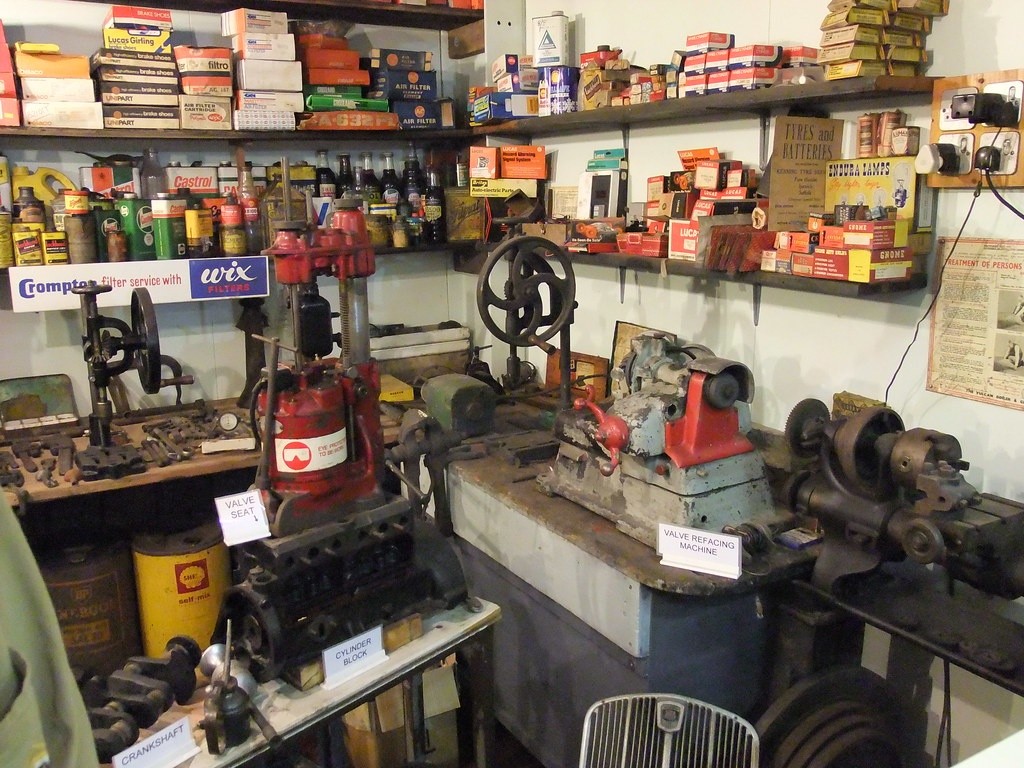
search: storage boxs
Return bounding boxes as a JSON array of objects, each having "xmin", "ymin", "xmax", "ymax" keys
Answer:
[
  {"xmin": 172, "ymin": 44, "xmax": 233, "ymax": 97},
  {"xmin": 469, "ymin": 145, "xmax": 501, "ymax": 179},
  {"xmin": 221, "ymin": 8, "xmax": 304, "ymax": 131},
  {"xmin": 577, "ymin": 148, "xmax": 628, "ymax": 218},
  {"xmin": 492, "ymin": 53, "xmax": 539, "ymax": 91},
  {"xmin": 21, "ymin": 100, "xmax": 105, "ymax": 129},
  {"xmin": 470, "ymin": 178, "xmax": 537, "ymax": 198},
  {"xmin": 218, "ymin": 167, "xmax": 267, "ymax": 198},
  {"xmin": 611, "ymin": 32, "xmax": 823, "ymax": 108},
  {"xmin": 20, "ymin": 78, "xmax": 96, "ymax": 101},
  {"xmin": 89, "ymin": 5, "xmax": 179, "ymax": 129},
  {"xmin": 816, "ymin": 0, "xmax": 949, "ymax": 81},
  {"xmin": 582, "ymin": 70, "xmax": 609, "ymax": 110},
  {"xmin": 761, "ymin": 156, "xmax": 931, "ymax": 285},
  {"xmin": 296, "ymin": 34, "xmax": 399, "ymax": 129},
  {"xmin": 370, "ymin": 324, "xmax": 471, "ymax": 382},
  {"xmin": 266, "ymin": 166, "xmax": 317, "ymax": 197},
  {"xmin": 521, "ymin": 220, "xmax": 596, "ymax": 245},
  {"xmin": 580, "ymin": 48, "xmax": 622, "ymax": 69},
  {"xmin": 179, "ymin": 94, "xmax": 232, "ymax": 130},
  {"xmin": 0, "ymin": 0, "xmax": 943, "ymax": 325},
  {"xmin": 616, "ymin": 147, "xmax": 757, "ymax": 261},
  {"xmin": 474, "ymin": 92, "xmax": 538, "ymax": 124},
  {"xmin": 328, "ymin": 653, "xmax": 461, "ymax": 768},
  {"xmin": 0, "ymin": 18, "xmax": 20, "ymax": 127},
  {"xmin": 78, "ymin": 166, "xmax": 219, "ymax": 199},
  {"xmin": 361, "ymin": 49, "xmax": 455, "ymax": 129},
  {"xmin": 396, "ymin": 0, "xmax": 484, "ymax": 9},
  {"xmin": 548, "ymin": 186, "xmax": 577, "ymax": 219},
  {"xmin": 15, "ymin": 51, "xmax": 90, "ymax": 78}
]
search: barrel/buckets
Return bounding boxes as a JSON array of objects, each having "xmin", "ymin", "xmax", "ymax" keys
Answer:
[
  {"xmin": 130, "ymin": 513, "xmax": 235, "ymax": 688},
  {"xmin": 33, "ymin": 533, "xmax": 143, "ymax": 683}
]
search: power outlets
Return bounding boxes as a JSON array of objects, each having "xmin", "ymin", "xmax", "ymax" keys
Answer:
[
  {"xmin": 940, "ymin": 87, "xmax": 978, "ymax": 130},
  {"xmin": 937, "ymin": 133, "xmax": 974, "ymax": 176},
  {"xmin": 977, "ymin": 130, "xmax": 1021, "ymax": 175}
]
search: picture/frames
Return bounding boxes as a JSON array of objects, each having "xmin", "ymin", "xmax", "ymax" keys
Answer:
[{"xmin": 926, "ymin": 237, "xmax": 1024, "ymax": 412}]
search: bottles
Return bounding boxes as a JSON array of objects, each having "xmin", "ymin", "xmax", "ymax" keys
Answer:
[
  {"xmin": 307, "ymin": 147, "xmax": 472, "ymax": 249},
  {"xmin": 0, "ymin": 146, "xmax": 306, "ymax": 274}
]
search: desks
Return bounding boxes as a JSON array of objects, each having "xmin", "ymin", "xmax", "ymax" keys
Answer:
[{"xmin": 136, "ymin": 596, "xmax": 502, "ymax": 768}]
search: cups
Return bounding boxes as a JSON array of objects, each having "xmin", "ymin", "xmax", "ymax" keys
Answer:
[
  {"xmin": 107, "ymin": 230, "xmax": 128, "ymax": 262},
  {"xmin": 64, "ymin": 190, "xmax": 89, "ymax": 215},
  {"xmin": 311, "ymin": 195, "xmax": 333, "ymax": 230}
]
